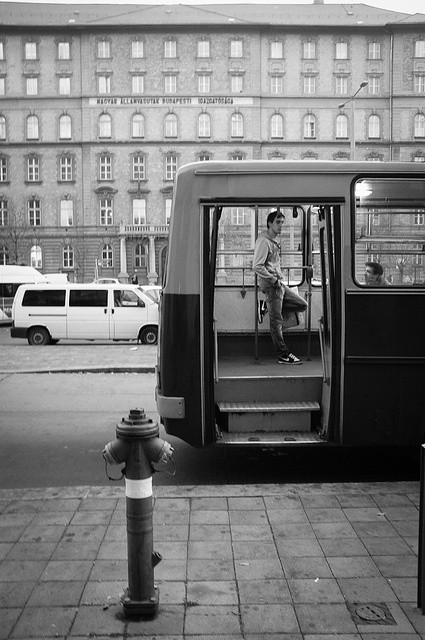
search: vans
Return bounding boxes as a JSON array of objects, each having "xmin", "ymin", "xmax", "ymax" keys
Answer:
[
  {"xmin": 121, "ymin": 285, "xmax": 162, "ymax": 306},
  {"xmin": 10, "ymin": 284, "xmax": 159, "ymax": 346}
]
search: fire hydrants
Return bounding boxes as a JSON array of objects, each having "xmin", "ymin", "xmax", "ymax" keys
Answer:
[{"xmin": 101, "ymin": 408, "xmax": 176, "ymax": 621}]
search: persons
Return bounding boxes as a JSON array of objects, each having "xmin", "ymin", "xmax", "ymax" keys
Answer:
[
  {"xmin": 363, "ymin": 260, "xmax": 393, "ymax": 287},
  {"xmin": 252, "ymin": 212, "xmax": 307, "ymax": 365}
]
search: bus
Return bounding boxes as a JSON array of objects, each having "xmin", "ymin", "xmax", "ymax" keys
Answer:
[
  {"xmin": 1, "ymin": 265, "xmax": 52, "ymax": 324},
  {"xmin": 155, "ymin": 160, "xmax": 425, "ymax": 454}
]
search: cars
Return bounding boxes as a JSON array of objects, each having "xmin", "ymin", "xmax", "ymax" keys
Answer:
[{"xmin": 91, "ymin": 277, "xmax": 121, "ymax": 284}]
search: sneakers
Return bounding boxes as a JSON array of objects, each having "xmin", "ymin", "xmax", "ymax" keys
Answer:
[
  {"xmin": 257, "ymin": 299, "xmax": 268, "ymax": 324},
  {"xmin": 276, "ymin": 352, "xmax": 303, "ymax": 365}
]
